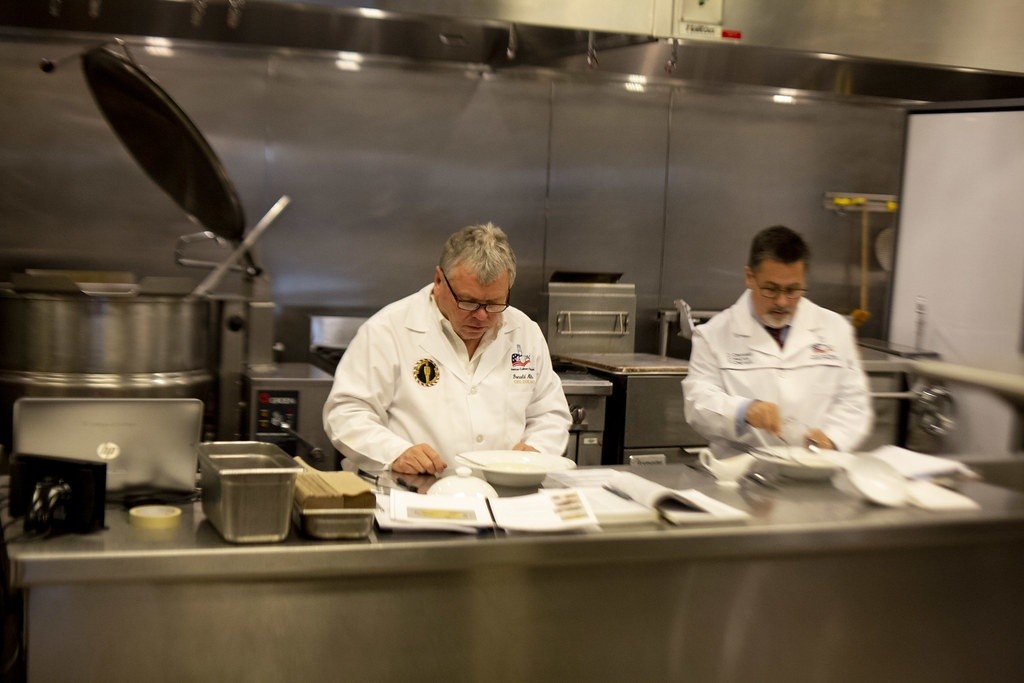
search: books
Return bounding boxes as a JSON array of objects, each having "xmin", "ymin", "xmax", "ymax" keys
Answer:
[{"xmin": 376, "ymin": 470, "xmax": 750, "ymax": 533}]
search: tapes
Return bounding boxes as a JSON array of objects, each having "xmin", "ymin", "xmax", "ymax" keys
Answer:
[{"xmin": 129, "ymin": 506, "xmax": 183, "ymax": 528}]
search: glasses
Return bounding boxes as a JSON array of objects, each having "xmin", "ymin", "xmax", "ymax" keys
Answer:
[
  {"xmin": 439, "ymin": 267, "xmax": 511, "ymax": 313},
  {"xmin": 751, "ymin": 268, "xmax": 809, "ymax": 299}
]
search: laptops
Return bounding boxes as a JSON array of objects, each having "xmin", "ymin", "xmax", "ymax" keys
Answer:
[{"xmin": 14, "ymin": 398, "xmax": 204, "ymax": 493}]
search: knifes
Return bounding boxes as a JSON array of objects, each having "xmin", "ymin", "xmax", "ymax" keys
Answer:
[{"xmin": 357, "ymin": 468, "xmax": 397, "ymax": 488}]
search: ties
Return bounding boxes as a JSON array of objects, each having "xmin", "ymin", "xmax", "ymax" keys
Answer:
[{"xmin": 763, "ymin": 325, "xmax": 785, "ymax": 348}]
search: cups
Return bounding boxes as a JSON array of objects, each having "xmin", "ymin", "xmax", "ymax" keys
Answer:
[{"xmin": 698, "ymin": 449, "xmax": 754, "ymax": 487}]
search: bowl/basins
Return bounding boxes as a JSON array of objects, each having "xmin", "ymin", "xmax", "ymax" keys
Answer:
[
  {"xmin": 454, "ymin": 450, "xmax": 576, "ymax": 486},
  {"xmin": 750, "ymin": 445, "xmax": 839, "ymax": 481}
]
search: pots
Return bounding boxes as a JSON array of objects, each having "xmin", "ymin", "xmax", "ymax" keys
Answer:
[{"xmin": 0, "ymin": 290, "xmax": 220, "ymax": 472}]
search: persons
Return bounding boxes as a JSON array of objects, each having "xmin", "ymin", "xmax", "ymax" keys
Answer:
[
  {"xmin": 682, "ymin": 226, "xmax": 875, "ymax": 460},
  {"xmin": 321, "ymin": 220, "xmax": 575, "ymax": 481}
]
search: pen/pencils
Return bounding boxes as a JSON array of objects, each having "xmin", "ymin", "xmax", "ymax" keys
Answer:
[{"xmin": 602, "ymin": 483, "xmax": 629, "ymax": 502}]
